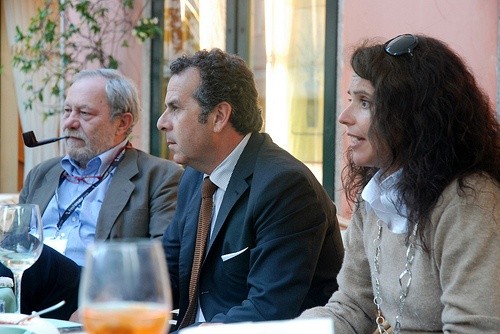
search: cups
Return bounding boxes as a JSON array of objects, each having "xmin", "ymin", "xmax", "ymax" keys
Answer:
[{"xmin": 78, "ymin": 238, "xmax": 172, "ymax": 334}]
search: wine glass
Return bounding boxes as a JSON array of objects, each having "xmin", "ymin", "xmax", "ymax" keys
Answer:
[{"xmin": 0, "ymin": 203, "xmax": 44, "ymax": 314}]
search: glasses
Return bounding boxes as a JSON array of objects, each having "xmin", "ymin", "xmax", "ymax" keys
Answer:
[
  {"xmin": 384, "ymin": 33, "xmax": 418, "ymax": 62},
  {"xmin": 62, "ymin": 145, "xmax": 130, "ymax": 185}
]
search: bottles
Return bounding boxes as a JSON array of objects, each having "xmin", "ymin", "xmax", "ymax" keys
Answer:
[{"xmin": 0, "ymin": 276, "xmax": 16, "ymax": 314}]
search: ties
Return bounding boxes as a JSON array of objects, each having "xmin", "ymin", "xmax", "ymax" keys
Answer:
[{"xmin": 172, "ymin": 176, "xmax": 219, "ymax": 330}]
